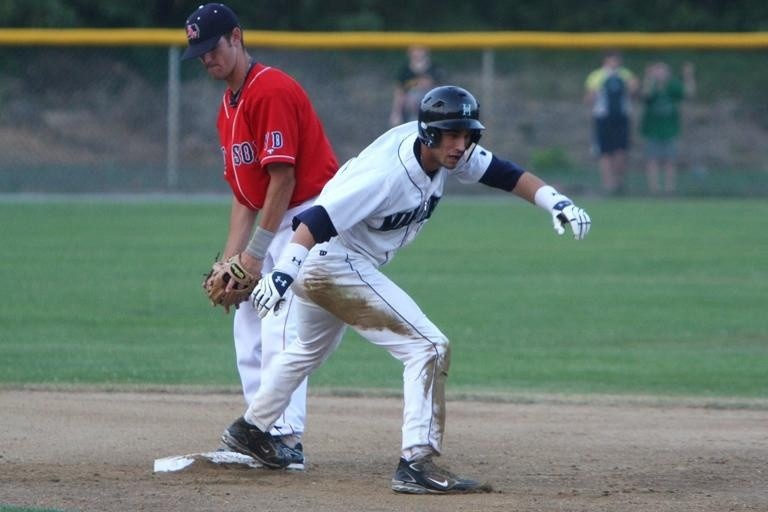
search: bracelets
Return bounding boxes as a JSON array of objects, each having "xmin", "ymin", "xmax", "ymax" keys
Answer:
[{"xmin": 243, "ymin": 224, "xmax": 274, "ymax": 261}]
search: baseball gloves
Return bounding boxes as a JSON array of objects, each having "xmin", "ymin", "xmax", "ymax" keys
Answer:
[{"xmin": 204, "ymin": 254, "xmax": 261, "ymax": 307}]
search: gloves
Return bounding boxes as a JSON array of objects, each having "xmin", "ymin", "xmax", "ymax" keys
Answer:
[
  {"xmin": 250, "ymin": 243, "xmax": 310, "ymax": 319},
  {"xmin": 534, "ymin": 186, "xmax": 591, "ymax": 240}
]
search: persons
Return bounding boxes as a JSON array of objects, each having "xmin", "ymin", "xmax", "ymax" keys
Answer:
[
  {"xmin": 636, "ymin": 58, "xmax": 696, "ymax": 198},
  {"xmin": 581, "ymin": 49, "xmax": 642, "ymax": 198},
  {"xmin": 384, "ymin": 45, "xmax": 445, "ymax": 126},
  {"xmin": 215, "ymin": 82, "xmax": 592, "ymax": 498},
  {"xmin": 176, "ymin": 1, "xmax": 342, "ymax": 473}
]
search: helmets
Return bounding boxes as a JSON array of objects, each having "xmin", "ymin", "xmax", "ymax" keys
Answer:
[{"xmin": 418, "ymin": 85, "xmax": 487, "ymax": 149}]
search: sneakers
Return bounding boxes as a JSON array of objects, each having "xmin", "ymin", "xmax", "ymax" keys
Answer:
[
  {"xmin": 391, "ymin": 454, "xmax": 481, "ymax": 494},
  {"xmin": 208, "ymin": 415, "xmax": 305, "ymax": 471}
]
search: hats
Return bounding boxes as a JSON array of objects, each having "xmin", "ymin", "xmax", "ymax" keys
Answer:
[{"xmin": 180, "ymin": 2, "xmax": 240, "ymax": 62}]
